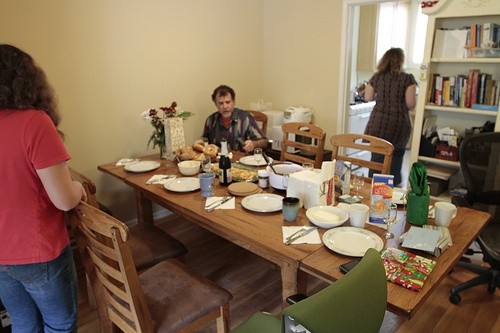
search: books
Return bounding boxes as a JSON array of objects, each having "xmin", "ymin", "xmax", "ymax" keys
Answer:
[
  {"xmin": 430, "ymin": 69, "xmax": 500, "ymax": 108},
  {"xmin": 471, "ymin": 22, "xmax": 500, "ymax": 49}
]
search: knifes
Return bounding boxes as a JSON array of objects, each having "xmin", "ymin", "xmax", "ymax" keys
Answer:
[
  {"xmin": 285, "ymin": 226, "xmax": 318, "ymax": 245},
  {"xmin": 207, "ymin": 196, "xmax": 232, "ymax": 212}
]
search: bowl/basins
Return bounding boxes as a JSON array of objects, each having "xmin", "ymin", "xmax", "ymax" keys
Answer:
[{"xmin": 178, "ymin": 160, "xmax": 200, "ymax": 176}]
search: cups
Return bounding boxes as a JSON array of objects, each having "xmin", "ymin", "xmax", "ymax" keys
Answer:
[
  {"xmin": 198, "ymin": 173, "xmax": 216, "ymax": 197},
  {"xmin": 349, "ymin": 203, "xmax": 369, "ymax": 228},
  {"xmin": 201, "ymin": 155, "xmax": 211, "ymax": 173},
  {"xmin": 266, "ymin": 162, "xmax": 303, "ymax": 190},
  {"xmin": 300, "ymin": 157, "xmax": 314, "ymax": 171},
  {"xmin": 434, "ymin": 202, "xmax": 458, "ymax": 227},
  {"xmin": 282, "ymin": 197, "xmax": 300, "ymax": 222}
]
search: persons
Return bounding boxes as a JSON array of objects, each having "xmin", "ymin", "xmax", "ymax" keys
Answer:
[
  {"xmin": 363, "ymin": 48, "xmax": 417, "ymax": 186},
  {"xmin": 203, "ymin": 86, "xmax": 266, "ymax": 153},
  {"xmin": 0, "ymin": 44, "xmax": 89, "ymax": 333}
]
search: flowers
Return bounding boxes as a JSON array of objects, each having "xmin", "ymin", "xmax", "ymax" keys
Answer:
[{"xmin": 146, "ymin": 101, "xmax": 195, "ymax": 148}]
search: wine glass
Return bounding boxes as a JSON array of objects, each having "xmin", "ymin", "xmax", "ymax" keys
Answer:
[
  {"xmin": 253, "ymin": 148, "xmax": 263, "ymax": 173},
  {"xmin": 353, "ymin": 172, "xmax": 365, "ymax": 201},
  {"xmin": 383, "ymin": 202, "xmax": 397, "ymax": 239}
]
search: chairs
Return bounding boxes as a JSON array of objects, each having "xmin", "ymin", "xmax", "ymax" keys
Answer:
[
  {"xmin": 280, "ymin": 122, "xmax": 327, "ymax": 169},
  {"xmin": 449, "ymin": 131, "xmax": 500, "ymax": 305},
  {"xmin": 233, "ymin": 248, "xmax": 388, "ymax": 333},
  {"xmin": 330, "ymin": 134, "xmax": 396, "ymax": 183},
  {"xmin": 67, "ymin": 168, "xmax": 186, "ymax": 308},
  {"xmin": 246, "ymin": 110, "xmax": 267, "ymax": 137},
  {"xmin": 76, "ymin": 202, "xmax": 233, "ymax": 333}
]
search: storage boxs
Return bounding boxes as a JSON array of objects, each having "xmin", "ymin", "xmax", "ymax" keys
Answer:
[
  {"xmin": 334, "ymin": 161, "xmax": 352, "ymax": 198},
  {"xmin": 370, "ymin": 173, "xmax": 395, "ymax": 224}
]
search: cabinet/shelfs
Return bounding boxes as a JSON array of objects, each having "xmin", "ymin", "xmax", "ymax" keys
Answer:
[{"xmin": 409, "ymin": 0, "xmax": 500, "ymax": 201}]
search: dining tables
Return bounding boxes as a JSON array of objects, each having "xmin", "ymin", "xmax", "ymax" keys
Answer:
[{"xmin": 97, "ymin": 150, "xmax": 492, "ymax": 312}]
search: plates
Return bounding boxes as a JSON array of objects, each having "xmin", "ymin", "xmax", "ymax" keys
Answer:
[
  {"xmin": 164, "ymin": 176, "xmax": 201, "ymax": 192},
  {"xmin": 241, "ymin": 193, "xmax": 285, "ymax": 213},
  {"xmin": 306, "ymin": 206, "xmax": 350, "ymax": 228},
  {"xmin": 124, "ymin": 161, "xmax": 160, "ymax": 172},
  {"xmin": 228, "ymin": 182, "xmax": 263, "ymax": 197},
  {"xmin": 322, "ymin": 226, "xmax": 384, "ymax": 257},
  {"xmin": 392, "ymin": 187, "xmax": 409, "ymax": 204},
  {"xmin": 240, "ymin": 155, "xmax": 272, "ymax": 165}
]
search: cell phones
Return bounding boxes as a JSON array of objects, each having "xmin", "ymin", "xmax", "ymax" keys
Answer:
[{"xmin": 339, "ymin": 257, "xmax": 363, "ymax": 275}]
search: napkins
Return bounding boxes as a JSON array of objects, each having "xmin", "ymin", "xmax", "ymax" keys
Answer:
[{"xmin": 406, "ymin": 161, "xmax": 430, "ymax": 225}]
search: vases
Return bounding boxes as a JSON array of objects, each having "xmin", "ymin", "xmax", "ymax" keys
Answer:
[{"xmin": 160, "ymin": 144, "xmax": 166, "ymax": 159}]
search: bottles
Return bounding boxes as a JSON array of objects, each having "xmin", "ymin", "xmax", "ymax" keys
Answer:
[{"xmin": 218, "ymin": 139, "xmax": 232, "ymax": 187}]
